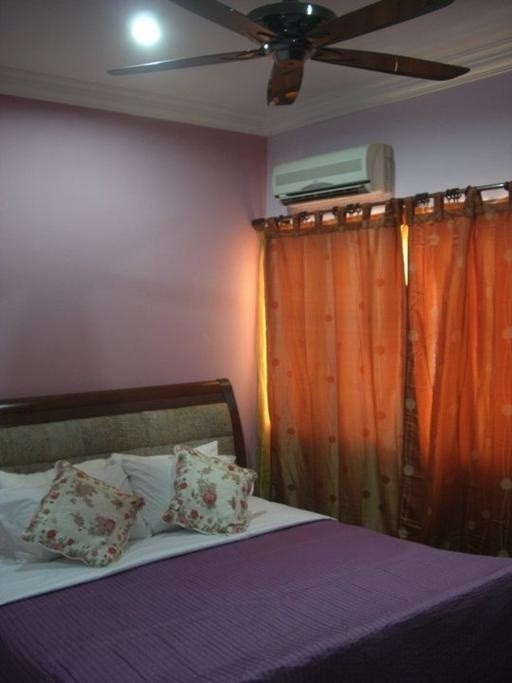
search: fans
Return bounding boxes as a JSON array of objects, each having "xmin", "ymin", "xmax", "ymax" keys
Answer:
[{"xmin": 107, "ymin": 0, "xmax": 471, "ymax": 109}]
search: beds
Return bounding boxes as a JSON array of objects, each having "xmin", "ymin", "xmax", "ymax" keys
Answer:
[{"xmin": 2, "ymin": 376, "xmax": 510, "ymax": 681}]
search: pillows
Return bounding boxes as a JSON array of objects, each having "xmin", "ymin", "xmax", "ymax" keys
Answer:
[{"xmin": 1, "ymin": 440, "xmax": 259, "ymax": 568}]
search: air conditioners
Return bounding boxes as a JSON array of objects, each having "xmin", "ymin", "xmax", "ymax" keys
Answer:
[{"xmin": 271, "ymin": 143, "xmax": 391, "ymax": 201}]
